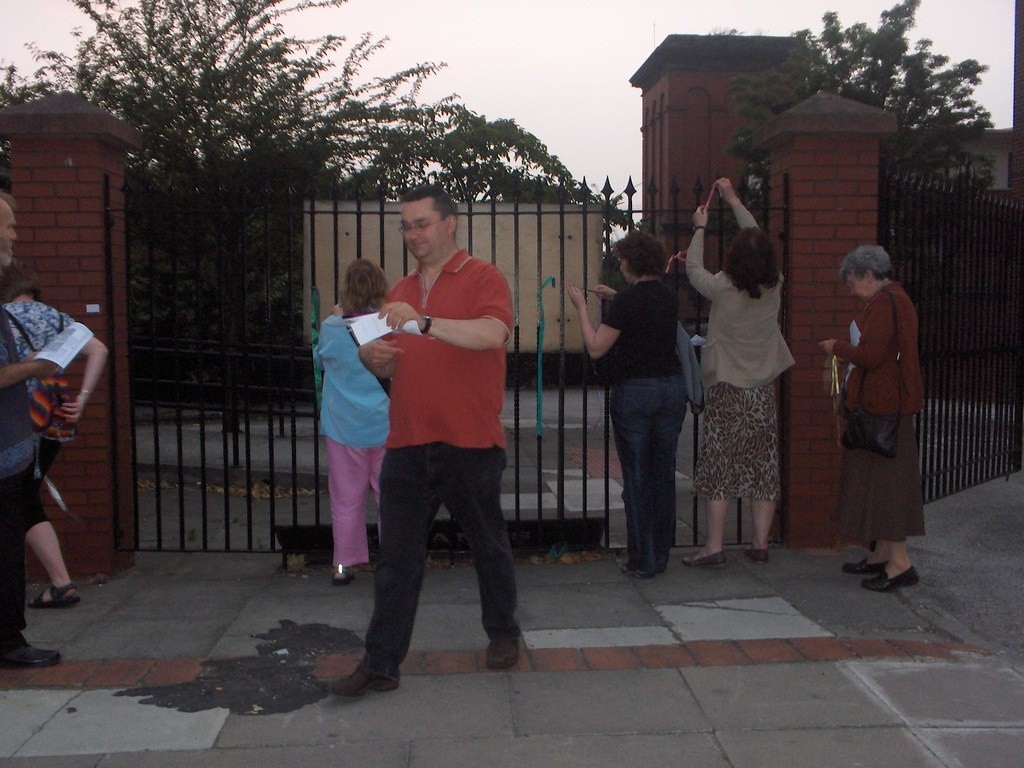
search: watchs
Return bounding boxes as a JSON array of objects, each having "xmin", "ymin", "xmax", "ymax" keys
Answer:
[{"xmin": 420, "ymin": 314, "xmax": 432, "ymax": 333}]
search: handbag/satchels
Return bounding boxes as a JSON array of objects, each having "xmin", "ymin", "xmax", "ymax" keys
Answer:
[{"xmin": 839, "ymin": 401, "xmax": 900, "ymax": 459}]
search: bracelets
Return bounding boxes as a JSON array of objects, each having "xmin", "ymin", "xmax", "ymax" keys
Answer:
[
  {"xmin": 692, "ymin": 225, "xmax": 705, "ymax": 235},
  {"xmin": 81, "ymin": 388, "xmax": 93, "ymax": 394}
]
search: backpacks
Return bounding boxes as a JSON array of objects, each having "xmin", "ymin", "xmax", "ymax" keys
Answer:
[{"xmin": 4, "ymin": 309, "xmax": 78, "ymax": 450}]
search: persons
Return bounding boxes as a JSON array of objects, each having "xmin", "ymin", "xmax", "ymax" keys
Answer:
[
  {"xmin": 332, "ymin": 184, "xmax": 521, "ymax": 697},
  {"xmin": 0, "ymin": 191, "xmax": 110, "ymax": 668},
  {"xmin": 819, "ymin": 243, "xmax": 927, "ymax": 593},
  {"xmin": 567, "ymin": 230, "xmax": 688, "ymax": 580},
  {"xmin": 681, "ymin": 177, "xmax": 796, "ymax": 568},
  {"xmin": 313, "ymin": 259, "xmax": 392, "ymax": 585}
]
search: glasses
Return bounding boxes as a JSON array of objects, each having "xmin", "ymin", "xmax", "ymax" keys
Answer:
[
  {"xmin": 399, "ymin": 218, "xmax": 446, "ymax": 234},
  {"xmin": 845, "ymin": 272, "xmax": 865, "ymax": 290}
]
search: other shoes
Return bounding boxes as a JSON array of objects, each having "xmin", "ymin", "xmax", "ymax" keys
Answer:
[
  {"xmin": 485, "ymin": 636, "xmax": 519, "ymax": 668},
  {"xmin": 683, "ymin": 550, "xmax": 728, "ymax": 568},
  {"xmin": 744, "ymin": 549, "xmax": 768, "ymax": 562},
  {"xmin": 332, "ymin": 665, "xmax": 400, "ymax": 697},
  {"xmin": 333, "ymin": 563, "xmax": 354, "ymax": 585},
  {"xmin": 622, "ymin": 563, "xmax": 665, "ymax": 578}
]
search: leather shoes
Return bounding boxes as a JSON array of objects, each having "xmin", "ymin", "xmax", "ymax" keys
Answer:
[
  {"xmin": 0, "ymin": 647, "xmax": 61, "ymax": 668},
  {"xmin": 842, "ymin": 556, "xmax": 888, "ymax": 574},
  {"xmin": 861, "ymin": 564, "xmax": 918, "ymax": 592}
]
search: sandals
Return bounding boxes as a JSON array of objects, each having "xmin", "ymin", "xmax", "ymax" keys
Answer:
[{"xmin": 27, "ymin": 582, "xmax": 82, "ymax": 609}]
